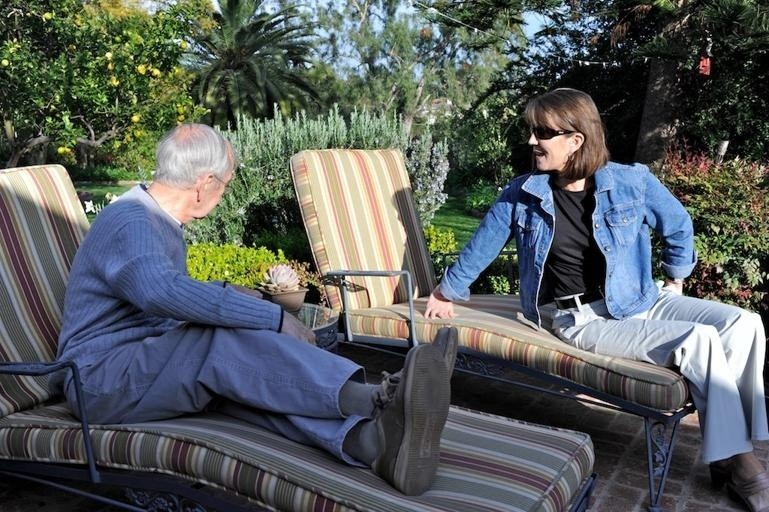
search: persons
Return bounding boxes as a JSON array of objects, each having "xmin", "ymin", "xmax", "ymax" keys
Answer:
[
  {"xmin": 421, "ymin": 86, "xmax": 769, "ymax": 511},
  {"xmin": 45, "ymin": 121, "xmax": 460, "ymax": 496}
]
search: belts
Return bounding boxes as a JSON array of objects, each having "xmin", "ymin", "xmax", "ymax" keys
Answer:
[{"xmin": 554, "ymin": 285, "xmax": 605, "ymax": 310}]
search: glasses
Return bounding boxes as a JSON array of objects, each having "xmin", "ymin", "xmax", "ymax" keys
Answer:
[
  {"xmin": 528, "ymin": 126, "xmax": 572, "ymax": 140},
  {"xmin": 214, "ymin": 174, "xmax": 234, "ymax": 195}
]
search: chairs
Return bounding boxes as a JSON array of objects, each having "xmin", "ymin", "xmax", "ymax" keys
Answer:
[
  {"xmin": 2, "ymin": 163, "xmax": 596, "ymax": 510},
  {"xmin": 288, "ymin": 149, "xmax": 696, "ymax": 510}
]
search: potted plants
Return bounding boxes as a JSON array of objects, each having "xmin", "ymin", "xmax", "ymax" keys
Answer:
[{"xmin": 256, "ymin": 262, "xmax": 309, "ymax": 312}]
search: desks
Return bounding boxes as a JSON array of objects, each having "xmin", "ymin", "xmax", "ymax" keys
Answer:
[{"xmin": 293, "ymin": 301, "xmax": 338, "ymax": 350}]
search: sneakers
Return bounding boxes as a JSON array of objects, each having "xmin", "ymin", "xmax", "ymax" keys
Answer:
[
  {"xmin": 370, "ymin": 344, "xmax": 452, "ymax": 496},
  {"xmin": 382, "ymin": 327, "xmax": 458, "ymax": 401}
]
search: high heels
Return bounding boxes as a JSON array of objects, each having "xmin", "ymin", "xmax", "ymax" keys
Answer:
[{"xmin": 709, "ymin": 459, "xmax": 769, "ymax": 512}]
